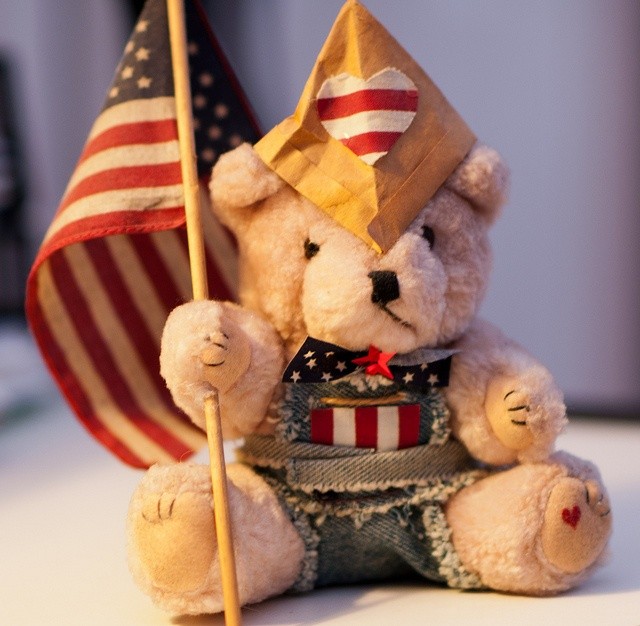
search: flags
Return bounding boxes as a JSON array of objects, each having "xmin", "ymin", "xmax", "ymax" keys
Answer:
[{"xmin": 25, "ymin": 0, "xmax": 267, "ymax": 475}]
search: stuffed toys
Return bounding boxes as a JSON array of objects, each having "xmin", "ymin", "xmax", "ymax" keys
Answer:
[{"xmin": 126, "ymin": 0, "xmax": 614, "ymax": 616}]
